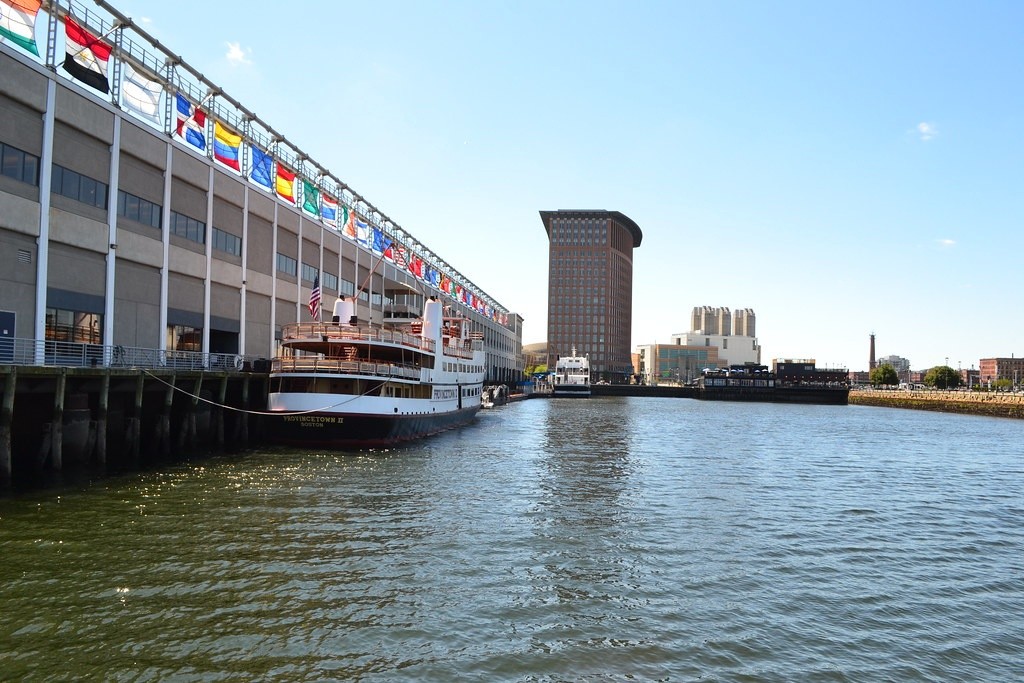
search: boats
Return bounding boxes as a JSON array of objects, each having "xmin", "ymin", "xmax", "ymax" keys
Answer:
[
  {"xmin": 553, "ymin": 346, "xmax": 591, "ymax": 399},
  {"xmin": 264, "ymin": 290, "xmax": 485, "ymax": 447}
]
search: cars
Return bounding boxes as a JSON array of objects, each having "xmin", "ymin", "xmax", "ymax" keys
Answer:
[
  {"xmin": 596, "ymin": 381, "xmax": 609, "ymax": 385},
  {"xmin": 850, "ymin": 382, "xmax": 1024, "ymax": 394}
]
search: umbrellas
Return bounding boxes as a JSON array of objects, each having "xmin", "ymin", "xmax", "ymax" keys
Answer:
[{"xmin": 703, "ymin": 366, "xmax": 768, "ymax": 374}]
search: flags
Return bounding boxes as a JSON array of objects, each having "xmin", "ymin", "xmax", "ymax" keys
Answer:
[
  {"xmin": 357, "ymin": 217, "xmax": 509, "ymax": 327},
  {"xmin": 251, "ymin": 144, "xmax": 274, "ymax": 189},
  {"xmin": 214, "ymin": 120, "xmax": 243, "ymax": 173},
  {"xmin": 62, "ymin": 14, "xmax": 116, "ymax": 96},
  {"xmin": 303, "ymin": 177, "xmax": 320, "ymax": 216},
  {"xmin": 1, "ymin": 0, "xmax": 42, "ymax": 57},
  {"xmin": 276, "ymin": 161, "xmax": 297, "ymax": 203},
  {"xmin": 341, "ymin": 204, "xmax": 356, "ymax": 237},
  {"xmin": 308, "ymin": 276, "xmax": 320, "ymax": 321},
  {"xmin": 322, "ymin": 194, "xmax": 338, "ymax": 222},
  {"xmin": 176, "ymin": 90, "xmax": 208, "ymax": 152},
  {"xmin": 119, "ymin": 60, "xmax": 164, "ymax": 127}
]
